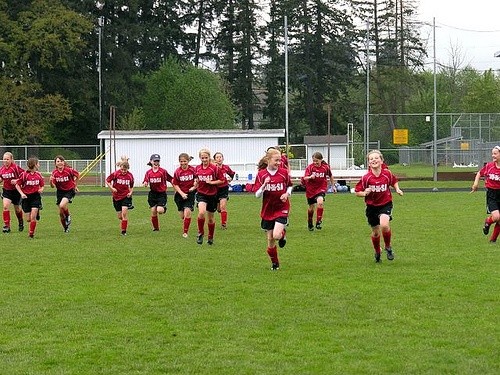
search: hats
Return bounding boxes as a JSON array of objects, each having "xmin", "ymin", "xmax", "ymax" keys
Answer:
[{"xmin": 150, "ymin": 154, "xmax": 160, "ymax": 161}]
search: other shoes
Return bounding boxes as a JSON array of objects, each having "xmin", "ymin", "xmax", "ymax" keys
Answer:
[{"xmin": 488, "ymin": 240, "xmax": 496, "ymax": 245}]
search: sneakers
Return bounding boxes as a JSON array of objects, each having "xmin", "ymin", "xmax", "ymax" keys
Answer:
[
  {"xmin": 30, "ymin": 233, "xmax": 33, "ymax": 237},
  {"xmin": 271, "ymin": 262, "xmax": 280, "ymax": 271},
  {"xmin": 316, "ymin": 221, "xmax": 322, "ymax": 230},
  {"xmin": 308, "ymin": 221, "xmax": 314, "ymax": 232},
  {"xmin": 152, "ymin": 228, "xmax": 159, "ymax": 232},
  {"xmin": 384, "ymin": 247, "xmax": 394, "ymax": 261},
  {"xmin": 124, "ymin": 233, "xmax": 127, "ymax": 235},
  {"xmin": 63, "ymin": 227, "xmax": 69, "ymax": 233},
  {"xmin": 208, "ymin": 239, "xmax": 213, "ymax": 245},
  {"xmin": 65, "ymin": 216, "xmax": 71, "ymax": 225},
  {"xmin": 279, "ymin": 228, "xmax": 287, "ymax": 248},
  {"xmin": 183, "ymin": 233, "xmax": 188, "ymax": 238},
  {"xmin": 483, "ymin": 217, "xmax": 490, "ymax": 236},
  {"xmin": 19, "ymin": 223, "xmax": 24, "ymax": 231},
  {"xmin": 374, "ymin": 254, "xmax": 382, "ymax": 264},
  {"xmin": 196, "ymin": 233, "xmax": 203, "ymax": 244},
  {"xmin": 37, "ymin": 216, "xmax": 40, "ymax": 220},
  {"xmin": 3, "ymin": 226, "xmax": 10, "ymax": 233},
  {"xmin": 220, "ymin": 224, "xmax": 226, "ymax": 230}
]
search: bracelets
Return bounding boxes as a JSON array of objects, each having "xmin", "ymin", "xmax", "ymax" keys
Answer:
[{"xmin": 332, "ymin": 186, "xmax": 335, "ymax": 189}]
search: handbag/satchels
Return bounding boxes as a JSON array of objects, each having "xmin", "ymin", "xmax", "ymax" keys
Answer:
[
  {"xmin": 292, "ymin": 184, "xmax": 306, "ymax": 192},
  {"xmin": 326, "ymin": 177, "xmax": 356, "ymax": 194},
  {"xmin": 227, "ymin": 185, "xmax": 243, "ymax": 192},
  {"xmin": 243, "ymin": 183, "xmax": 254, "ymax": 192}
]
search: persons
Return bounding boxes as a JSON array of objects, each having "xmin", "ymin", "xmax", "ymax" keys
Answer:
[
  {"xmin": 106, "ymin": 162, "xmax": 133, "ymax": 237},
  {"xmin": 195, "ymin": 148, "xmax": 225, "ymax": 245},
  {"xmin": 143, "ymin": 154, "xmax": 174, "ymax": 231},
  {"xmin": 15, "ymin": 158, "xmax": 45, "ymax": 238},
  {"xmin": 254, "ymin": 149, "xmax": 293, "ymax": 271},
  {"xmin": 259, "ymin": 146, "xmax": 290, "ymax": 226},
  {"xmin": 0, "ymin": 152, "xmax": 23, "ymax": 233},
  {"xmin": 470, "ymin": 146, "xmax": 500, "ymax": 243},
  {"xmin": 355, "ymin": 150, "xmax": 403, "ymax": 263},
  {"xmin": 174, "ymin": 153, "xmax": 197, "ymax": 237},
  {"xmin": 214, "ymin": 152, "xmax": 235, "ymax": 227},
  {"xmin": 50, "ymin": 155, "xmax": 79, "ymax": 232},
  {"xmin": 303, "ymin": 152, "xmax": 338, "ymax": 231},
  {"xmin": 117, "ymin": 155, "xmax": 135, "ymax": 209}
]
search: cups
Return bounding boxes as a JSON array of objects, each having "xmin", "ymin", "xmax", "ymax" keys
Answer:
[{"xmin": 249, "ymin": 174, "xmax": 252, "ymax": 180}]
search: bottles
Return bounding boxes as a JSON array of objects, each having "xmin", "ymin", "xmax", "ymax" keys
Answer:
[{"xmin": 235, "ymin": 174, "xmax": 238, "ymax": 180}]
